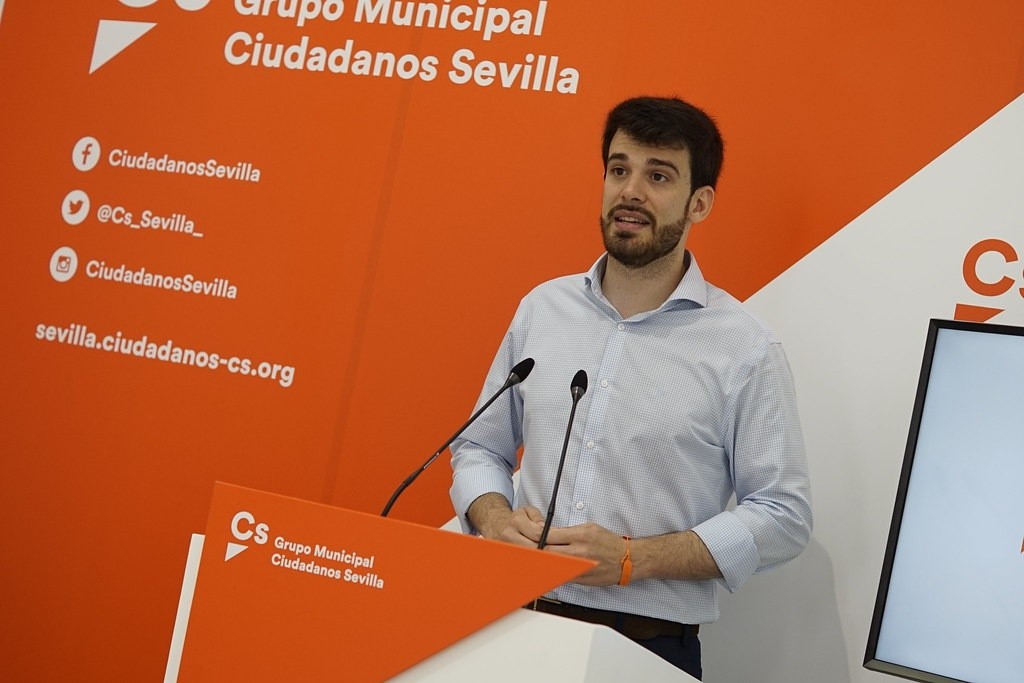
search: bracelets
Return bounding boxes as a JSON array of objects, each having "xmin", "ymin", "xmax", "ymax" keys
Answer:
[{"xmin": 618, "ymin": 535, "xmax": 633, "ymax": 587}]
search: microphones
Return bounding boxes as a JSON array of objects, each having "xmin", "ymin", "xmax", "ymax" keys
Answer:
[
  {"xmin": 538, "ymin": 370, "xmax": 587, "ymax": 549},
  {"xmin": 381, "ymin": 358, "xmax": 535, "ymax": 517}
]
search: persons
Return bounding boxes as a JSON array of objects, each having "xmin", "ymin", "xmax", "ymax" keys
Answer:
[{"xmin": 448, "ymin": 96, "xmax": 813, "ymax": 683}]
